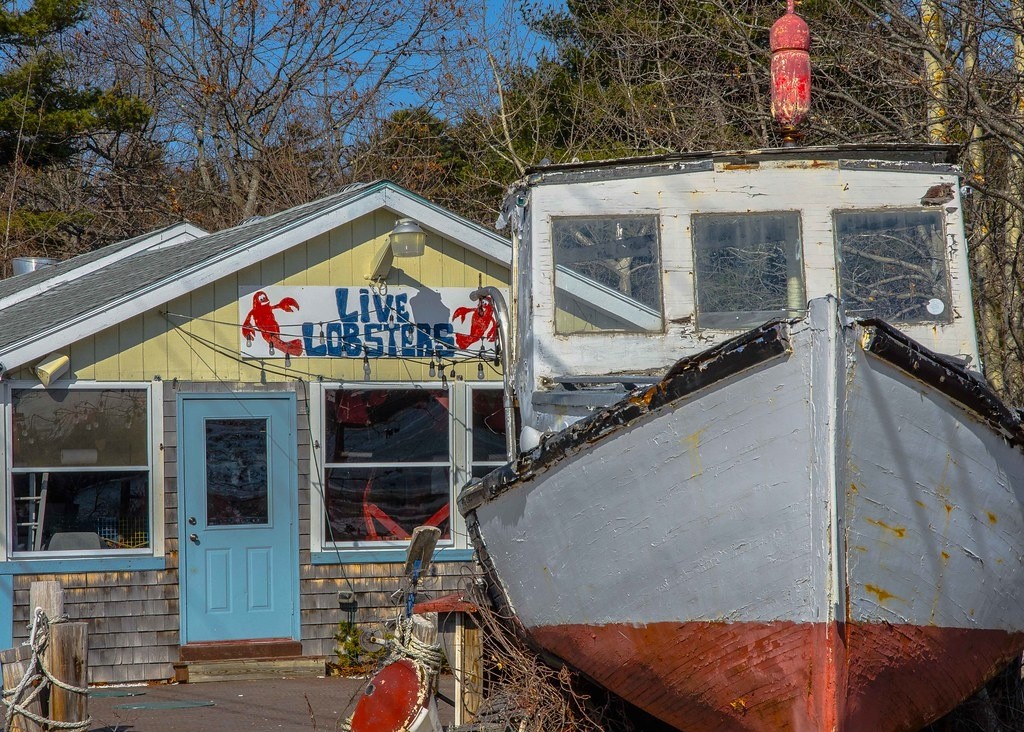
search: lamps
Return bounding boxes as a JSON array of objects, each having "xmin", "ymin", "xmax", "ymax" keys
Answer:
[
  {"xmin": 36, "ymin": 353, "xmax": 72, "ymax": 389},
  {"xmin": 368, "ymin": 219, "xmax": 427, "ymax": 292}
]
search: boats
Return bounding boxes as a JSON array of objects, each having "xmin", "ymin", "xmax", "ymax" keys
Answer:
[{"xmin": 456, "ymin": 0, "xmax": 1024, "ymax": 732}]
